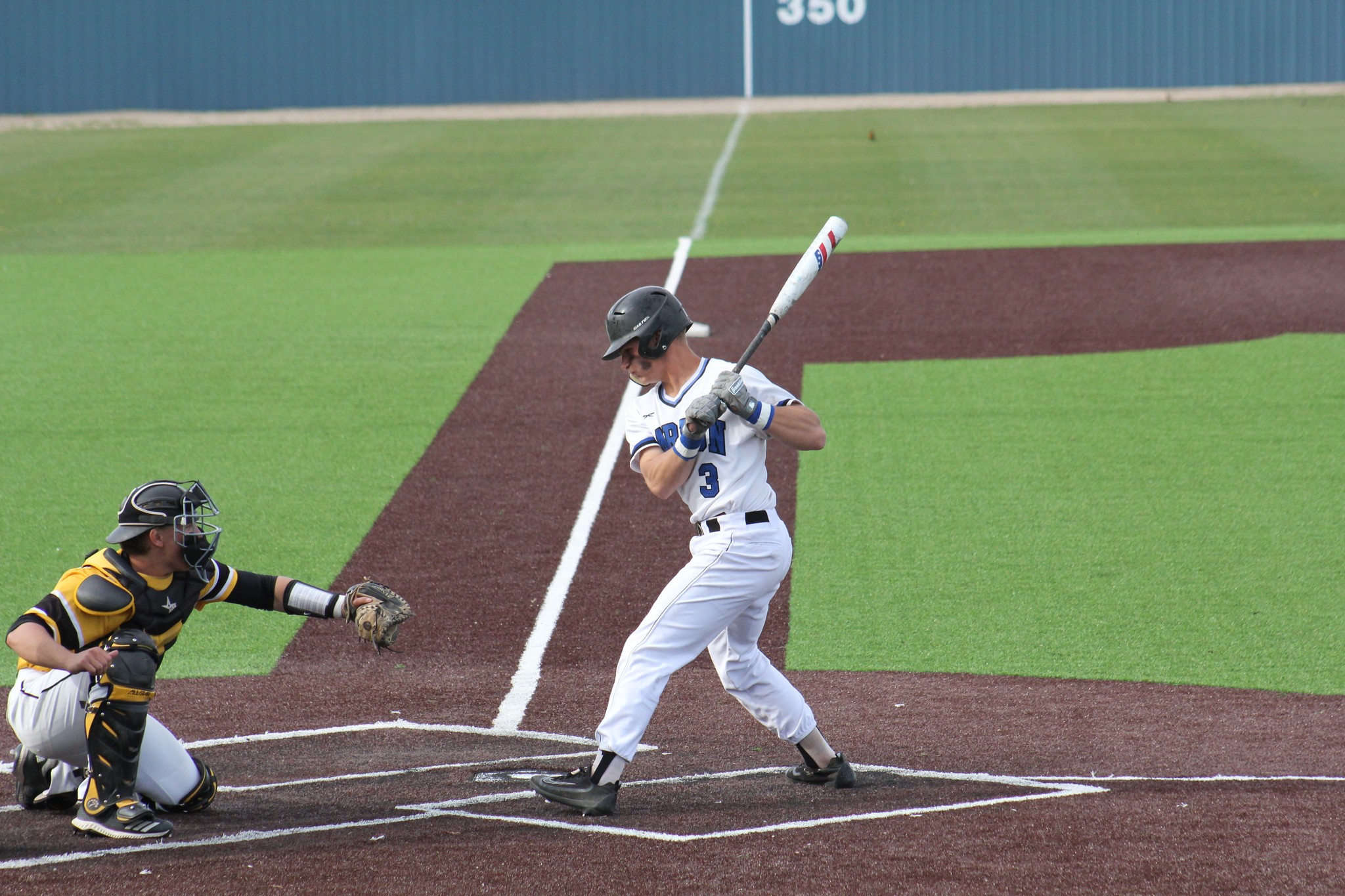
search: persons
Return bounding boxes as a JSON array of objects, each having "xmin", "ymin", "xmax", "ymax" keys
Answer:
[
  {"xmin": 531, "ymin": 286, "xmax": 857, "ymax": 815},
  {"xmin": 5, "ymin": 480, "xmax": 374, "ymax": 839}
]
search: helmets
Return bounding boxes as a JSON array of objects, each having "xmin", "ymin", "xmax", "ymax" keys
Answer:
[
  {"xmin": 602, "ymin": 286, "xmax": 693, "ymax": 361},
  {"xmin": 105, "ymin": 479, "xmax": 199, "ymax": 544}
]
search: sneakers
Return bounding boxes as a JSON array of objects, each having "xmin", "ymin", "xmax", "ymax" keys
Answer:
[
  {"xmin": 71, "ymin": 800, "xmax": 174, "ymax": 840},
  {"xmin": 12, "ymin": 743, "xmax": 59, "ymax": 809},
  {"xmin": 784, "ymin": 752, "xmax": 858, "ymax": 788},
  {"xmin": 529, "ymin": 763, "xmax": 622, "ymax": 816}
]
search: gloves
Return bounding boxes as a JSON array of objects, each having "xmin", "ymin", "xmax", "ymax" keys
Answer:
[
  {"xmin": 682, "ymin": 394, "xmax": 728, "ymax": 441},
  {"xmin": 710, "ymin": 371, "xmax": 758, "ymax": 420}
]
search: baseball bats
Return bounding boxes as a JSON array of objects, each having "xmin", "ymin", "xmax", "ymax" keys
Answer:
[{"xmin": 696, "ymin": 216, "xmax": 849, "ymax": 427}]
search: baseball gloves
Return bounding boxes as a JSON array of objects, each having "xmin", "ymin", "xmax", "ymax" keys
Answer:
[{"xmin": 344, "ymin": 580, "xmax": 413, "ymax": 648}]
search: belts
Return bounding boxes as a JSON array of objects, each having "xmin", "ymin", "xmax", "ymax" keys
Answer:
[{"xmin": 695, "ymin": 510, "xmax": 769, "ymax": 536}]
